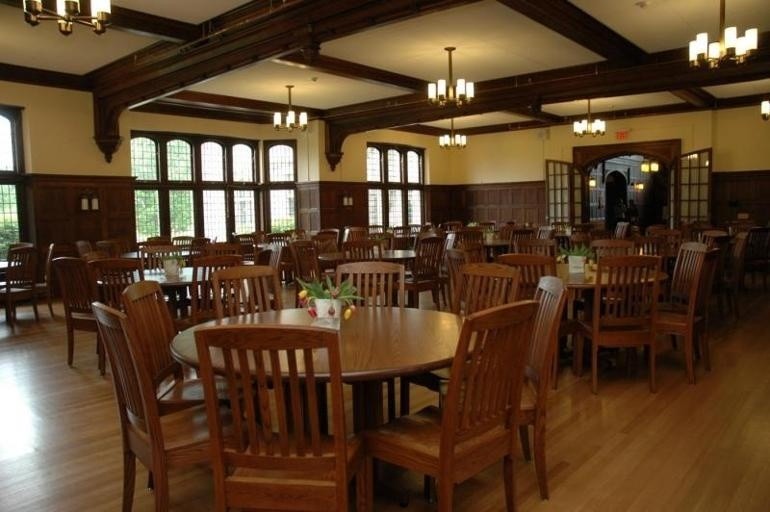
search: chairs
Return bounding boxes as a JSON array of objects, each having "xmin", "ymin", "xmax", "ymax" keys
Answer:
[
  {"xmin": 0, "ymin": 247, "xmax": 40, "ymax": 328},
  {"xmin": 614, "ymin": 220, "xmax": 630, "ymax": 239},
  {"xmin": 591, "ymin": 239, "xmax": 635, "ymax": 264},
  {"xmin": 290, "ymin": 240, "xmax": 335, "ymax": 307},
  {"xmin": 508, "ymin": 229, "xmax": 532, "ymax": 252},
  {"xmin": 538, "ymin": 226, "xmax": 556, "ymax": 238},
  {"xmin": 716, "ymin": 233, "xmax": 750, "ymax": 324},
  {"xmin": 345, "ymin": 227, "xmax": 366, "ymax": 239},
  {"xmin": 8, "ymin": 242, "xmax": 32, "ymax": 261},
  {"xmin": 191, "ymin": 253, "xmax": 243, "ymax": 323},
  {"xmin": 514, "ymin": 238, "xmax": 557, "ymax": 263},
  {"xmin": 580, "ymin": 254, "xmax": 663, "ymax": 394},
  {"xmin": 121, "ymin": 280, "xmax": 259, "ymax": 490},
  {"xmin": 362, "ymin": 299, "xmax": 542, "ymax": 512},
  {"xmin": 87, "ymin": 257, "xmax": 144, "ymax": 312},
  {"xmin": 91, "ymin": 300, "xmax": 256, "ymax": 511},
  {"xmin": 342, "ymin": 240, "xmax": 382, "ymax": 262},
  {"xmin": 202, "ymin": 243, "xmax": 243, "ymax": 256},
  {"xmin": 442, "ymin": 233, "xmax": 460, "ymax": 273},
  {"xmin": 234, "ymin": 233, "xmax": 256, "ymax": 261},
  {"xmin": 700, "ymin": 230, "xmax": 728, "ymax": 245},
  {"xmin": 194, "ymin": 325, "xmax": 361, "ymax": 512},
  {"xmin": 50, "ymin": 257, "xmax": 107, "ymax": 376},
  {"xmin": 95, "ymin": 239, "xmax": 119, "ymax": 257},
  {"xmin": 653, "ymin": 241, "xmax": 709, "ymax": 384},
  {"xmin": 140, "ymin": 244, "xmax": 182, "ymax": 268},
  {"xmin": 732, "ymin": 219, "xmax": 753, "ymax": 234},
  {"xmin": 24, "ymin": 244, "xmax": 56, "ymax": 319},
  {"xmin": 438, "ymin": 275, "xmax": 569, "ymax": 499},
  {"xmin": 398, "ymin": 261, "xmax": 522, "ymax": 417},
  {"xmin": 367, "ymin": 220, "xmax": 462, "ymax": 232},
  {"xmin": 748, "ymin": 225, "xmax": 769, "ymax": 247},
  {"xmin": 75, "ymin": 239, "xmax": 93, "ymax": 258},
  {"xmin": 268, "ymin": 243, "xmax": 283, "ymax": 271},
  {"xmin": 209, "ymin": 265, "xmax": 284, "ymax": 319},
  {"xmin": 264, "ymin": 232, "xmax": 286, "ymax": 239},
  {"xmin": 398, "ymin": 235, "xmax": 443, "ymax": 307},
  {"xmin": 171, "ymin": 235, "xmax": 192, "ymax": 247},
  {"xmin": 333, "ymin": 260, "xmax": 405, "ymax": 309},
  {"xmin": 496, "ymin": 253, "xmax": 584, "ymax": 390},
  {"xmin": 634, "ymin": 238, "xmax": 665, "ymax": 254},
  {"xmin": 319, "ymin": 225, "xmax": 340, "ymax": 245}
]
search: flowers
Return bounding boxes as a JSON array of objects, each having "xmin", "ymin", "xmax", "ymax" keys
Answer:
[{"xmin": 295, "ymin": 275, "xmax": 365, "ymax": 317}]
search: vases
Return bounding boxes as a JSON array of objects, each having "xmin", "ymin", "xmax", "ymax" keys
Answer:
[{"xmin": 314, "ymin": 297, "xmax": 343, "ymax": 320}]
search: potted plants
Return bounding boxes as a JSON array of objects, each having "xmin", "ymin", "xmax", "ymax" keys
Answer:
[
  {"xmin": 369, "ymin": 233, "xmax": 388, "ymax": 256},
  {"xmin": 162, "ymin": 256, "xmax": 185, "ymax": 274},
  {"xmin": 483, "ymin": 225, "xmax": 501, "ymax": 241},
  {"xmin": 556, "ymin": 243, "xmax": 595, "ymax": 273}
]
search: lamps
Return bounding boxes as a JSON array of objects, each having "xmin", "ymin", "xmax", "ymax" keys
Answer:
[
  {"xmin": 21, "ymin": 0, "xmax": 113, "ymax": 36},
  {"xmin": 342, "ymin": 191, "xmax": 354, "ymax": 208},
  {"xmin": 77, "ymin": 185, "xmax": 98, "ymax": 214},
  {"xmin": 639, "ymin": 160, "xmax": 659, "ymax": 174},
  {"xmin": 573, "ymin": 98, "xmax": 606, "ymax": 137},
  {"xmin": 760, "ymin": 100, "xmax": 770, "ymax": 121},
  {"xmin": 439, "ymin": 118, "xmax": 467, "ymax": 150},
  {"xmin": 427, "ymin": 47, "xmax": 475, "ymax": 109},
  {"xmin": 273, "ymin": 84, "xmax": 308, "ymax": 133},
  {"xmin": 688, "ymin": 0, "xmax": 759, "ymax": 73}
]
